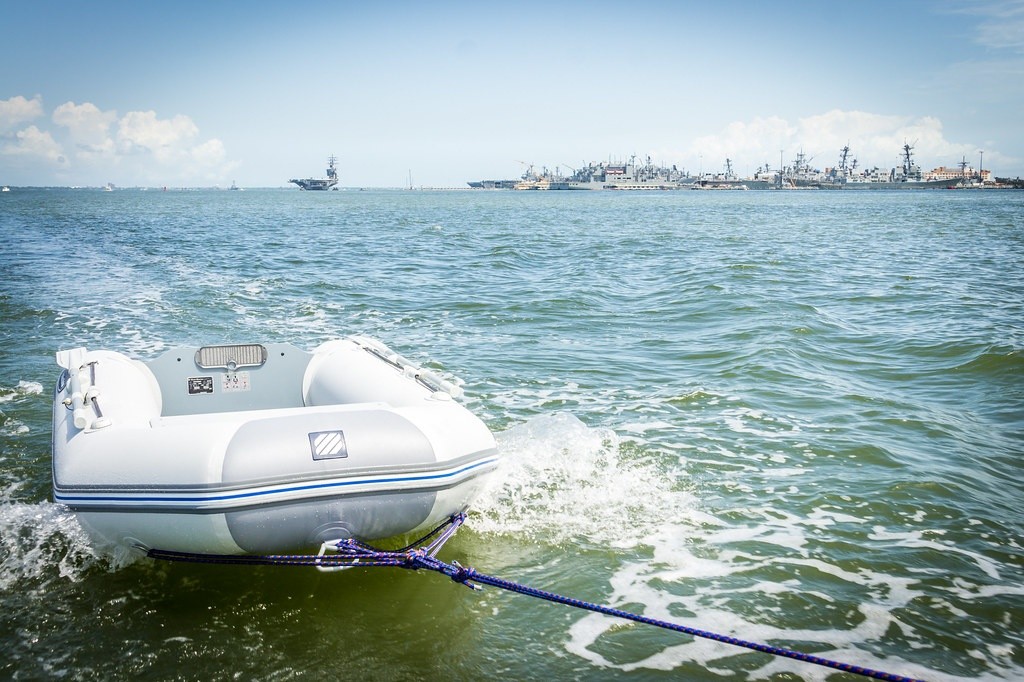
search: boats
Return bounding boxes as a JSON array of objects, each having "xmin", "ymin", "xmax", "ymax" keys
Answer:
[
  {"xmin": 49, "ymin": 335, "xmax": 501, "ymax": 560},
  {"xmin": 288, "ymin": 154, "xmax": 340, "ymax": 191},
  {"xmin": 465, "ymin": 144, "xmax": 1024, "ymax": 191}
]
